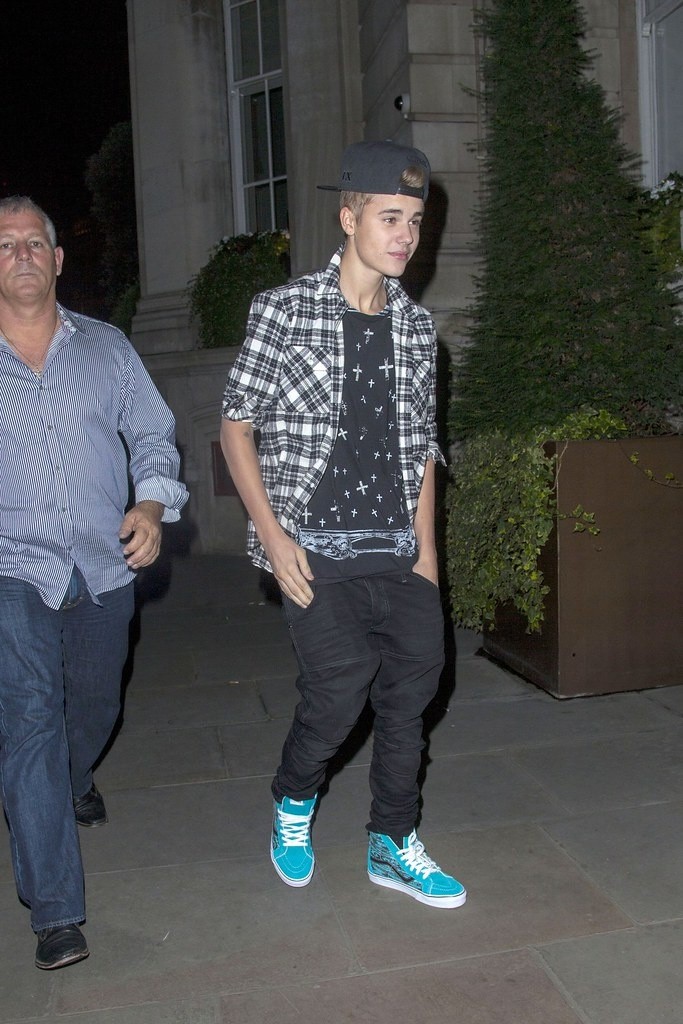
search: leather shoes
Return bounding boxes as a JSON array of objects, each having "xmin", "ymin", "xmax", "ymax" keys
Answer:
[
  {"xmin": 35, "ymin": 923, "xmax": 90, "ymax": 970},
  {"xmin": 72, "ymin": 781, "xmax": 108, "ymax": 829}
]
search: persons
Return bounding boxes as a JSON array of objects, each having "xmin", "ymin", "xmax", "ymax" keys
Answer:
[
  {"xmin": 217, "ymin": 140, "xmax": 467, "ymax": 909},
  {"xmin": 0, "ymin": 193, "xmax": 191, "ymax": 970}
]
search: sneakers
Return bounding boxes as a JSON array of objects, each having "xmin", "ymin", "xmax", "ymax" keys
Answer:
[
  {"xmin": 269, "ymin": 791, "xmax": 317, "ymax": 888},
  {"xmin": 367, "ymin": 829, "xmax": 467, "ymax": 908}
]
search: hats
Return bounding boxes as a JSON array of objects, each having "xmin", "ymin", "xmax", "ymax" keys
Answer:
[{"xmin": 316, "ymin": 139, "xmax": 430, "ymax": 201}]
